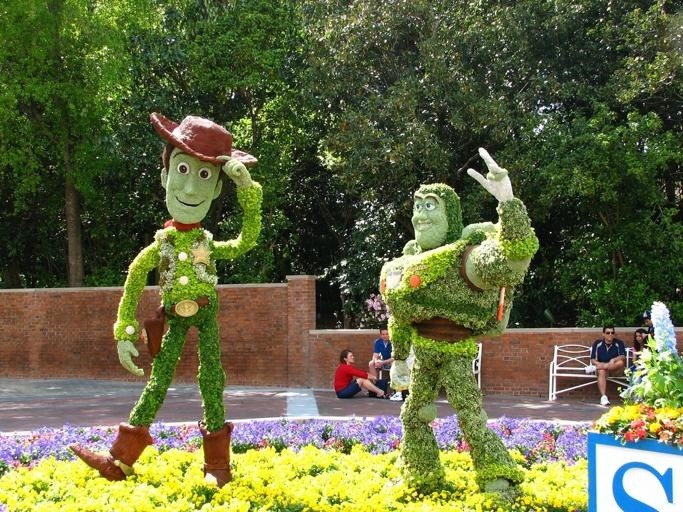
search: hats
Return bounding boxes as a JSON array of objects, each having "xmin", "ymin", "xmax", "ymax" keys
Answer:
[{"xmin": 643, "ymin": 310, "xmax": 651, "ymax": 318}]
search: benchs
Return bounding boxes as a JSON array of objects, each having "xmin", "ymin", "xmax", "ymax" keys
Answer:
[
  {"xmin": 379, "ymin": 342, "xmax": 482, "ymax": 390},
  {"xmin": 548, "ymin": 343, "xmax": 634, "ymax": 402}
]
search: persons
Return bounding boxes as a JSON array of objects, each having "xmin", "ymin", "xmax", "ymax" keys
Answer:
[
  {"xmin": 617, "ymin": 328, "xmax": 650, "ymax": 403},
  {"xmin": 584, "ymin": 323, "xmax": 626, "ymax": 407},
  {"xmin": 332, "ymin": 348, "xmax": 390, "ymax": 402},
  {"xmin": 366, "ymin": 325, "xmax": 393, "ymax": 380},
  {"xmin": 641, "ymin": 309, "xmax": 655, "ymax": 339},
  {"xmin": 390, "ymin": 341, "xmax": 414, "ymax": 403}
]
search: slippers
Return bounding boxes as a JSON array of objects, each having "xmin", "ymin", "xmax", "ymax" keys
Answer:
[{"xmin": 377, "ymin": 391, "xmax": 391, "ymax": 399}]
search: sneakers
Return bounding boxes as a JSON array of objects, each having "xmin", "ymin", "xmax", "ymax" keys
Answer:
[
  {"xmin": 619, "ymin": 388, "xmax": 632, "ymax": 398},
  {"xmin": 390, "ymin": 392, "xmax": 403, "ymax": 401},
  {"xmin": 600, "ymin": 395, "xmax": 610, "ymax": 405},
  {"xmin": 585, "ymin": 365, "xmax": 596, "ymax": 373}
]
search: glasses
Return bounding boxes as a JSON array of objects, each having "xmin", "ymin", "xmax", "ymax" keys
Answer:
[{"xmin": 606, "ymin": 332, "xmax": 614, "ymax": 334}]
